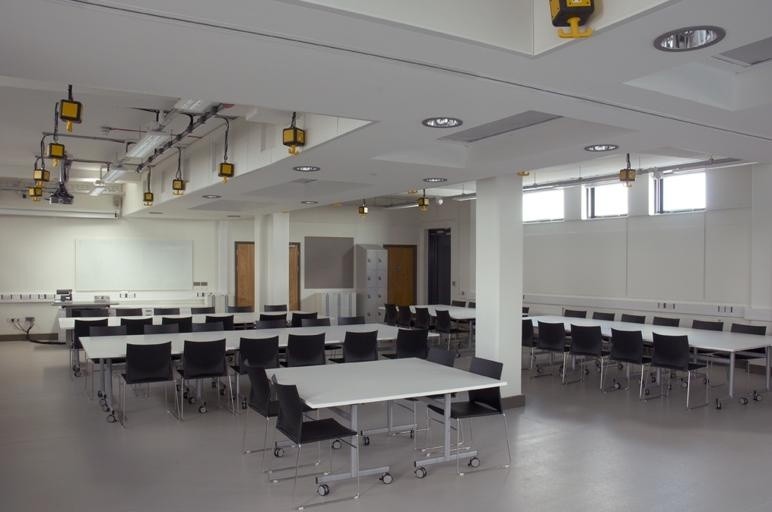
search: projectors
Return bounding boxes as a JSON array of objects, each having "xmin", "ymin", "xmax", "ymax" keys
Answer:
[{"xmin": 50, "ymin": 194, "xmax": 74, "ymax": 204}]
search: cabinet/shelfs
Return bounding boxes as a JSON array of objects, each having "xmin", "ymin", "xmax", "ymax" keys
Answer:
[{"xmin": 356, "ymin": 243, "xmax": 387, "ymax": 324}]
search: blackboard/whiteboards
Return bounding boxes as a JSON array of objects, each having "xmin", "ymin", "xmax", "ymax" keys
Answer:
[
  {"xmin": 75, "ymin": 240, "xmax": 193, "ymax": 292},
  {"xmin": 522, "ymin": 209, "xmax": 754, "ymax": 307}
]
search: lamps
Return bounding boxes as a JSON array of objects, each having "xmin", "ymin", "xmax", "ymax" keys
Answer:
[
  {"xmin": 142, "ymin": 165, "xmax": 154, "ymax": 206},
  {"xmin": 217, "ymin": 115, "xmax": 235, "ymax": 184},
  {"xmin": 358, "ymin": 199, "xmax": 368, "ymax": 218},
  {"xmin": 654, "ymin": 25, "xmax": 726, "ymax": 52},
  {"xmin": 549, "ymin": 0, "xmax": 594, "ymax": 39},
  {"xmin": 417, "ymin": 188, "xmax": 430, "ymax": 216},
  {"xmin": 282, "ymin": 112, "xmax": 305, "ymax": 160},
  {"xmin": 171, "ymin": 146, "xmax": 184, "ymax": 195},
  {"xmin": 619, "ymin": 154, "xmax": 637, "ymax": 186},
  {"xmin": 423, "ymin": 117, "xmax": 463, "ymax": 128},
  {"xmin": 22, "ymin": 84, "xmax": 83, "ymax": 206}
]
